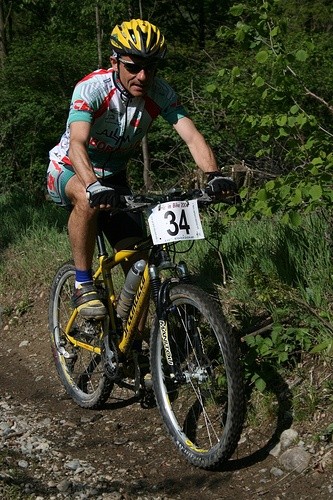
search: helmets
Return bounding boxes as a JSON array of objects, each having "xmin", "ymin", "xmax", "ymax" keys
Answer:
[{"xmin": 110, "ymin": 18, "xmax": 168, "ymax": 60}]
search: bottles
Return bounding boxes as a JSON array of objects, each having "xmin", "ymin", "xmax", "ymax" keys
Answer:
[{"xmin": 116, "ymin": 258, "xmax": 146, "ymax": 319}]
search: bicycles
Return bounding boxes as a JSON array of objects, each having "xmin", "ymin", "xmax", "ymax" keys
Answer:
[{"xmin": 47, "ymin": 190, "xmax": 246, "ymax": 469}]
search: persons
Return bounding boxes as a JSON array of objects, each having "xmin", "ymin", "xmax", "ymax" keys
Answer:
[{"xmin": 45, "ymin": 18, "xmax": 243, "ymax": 322}]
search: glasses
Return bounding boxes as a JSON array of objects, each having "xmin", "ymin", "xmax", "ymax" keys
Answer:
[{"xmin": 114, "ymin": 54, "xmax": 160, "ymax": 74}]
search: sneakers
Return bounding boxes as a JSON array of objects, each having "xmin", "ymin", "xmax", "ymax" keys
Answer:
[{"xmin": 70, "ymin": 281, "xmax": 108, "ymax": 321}]
